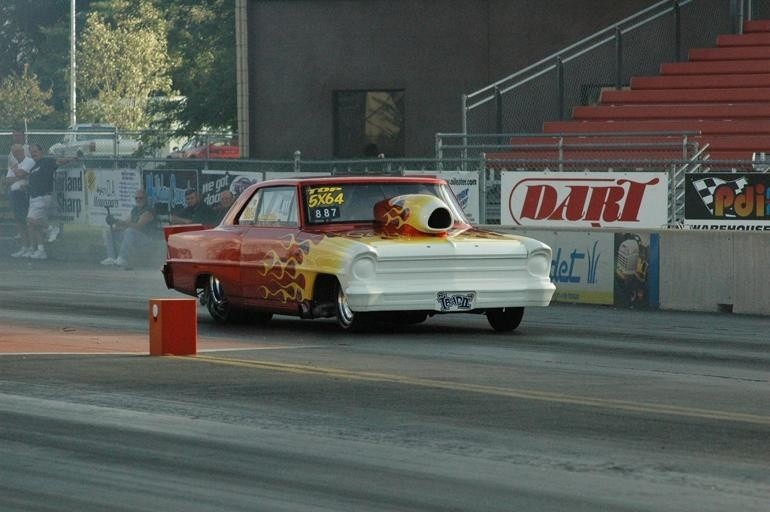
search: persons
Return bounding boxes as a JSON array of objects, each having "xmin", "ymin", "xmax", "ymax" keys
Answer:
[
  {"xmin": 178, "ymin": 189, "xmax": 236, "ymax": 224},
  {"xmin": 99, "ymin": 190, "xmax": 156, "ymax": 266},
  {"xmin": 6, "ymin": 128, "xmax": 78, "ymax": 260}
]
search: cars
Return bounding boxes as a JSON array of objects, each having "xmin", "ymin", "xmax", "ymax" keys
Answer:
[
  {"xmin": 166, "ymin": 133, "xmax": 239, "ymax": 158},
  {"xmin": 160, "ymin": 175, "xmax": 557, "ymax": 334}
]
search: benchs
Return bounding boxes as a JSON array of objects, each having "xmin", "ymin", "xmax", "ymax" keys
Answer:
[{"xmin": 477, "ymin": 19, "xmax": 769, "ymax": 171}]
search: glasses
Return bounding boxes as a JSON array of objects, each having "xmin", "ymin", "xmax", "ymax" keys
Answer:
[{"xmin": 135, "ymin": 196, "xmax": 144, "ymax": 200}]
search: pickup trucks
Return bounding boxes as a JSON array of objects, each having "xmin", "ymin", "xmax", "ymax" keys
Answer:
[{"xmin": 48, "ymin": 123, "xmax": 142, "ymax": 156}]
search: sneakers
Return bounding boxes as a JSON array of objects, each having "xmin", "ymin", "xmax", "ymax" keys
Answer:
[
  {"xmin": 47, "ymin": 226, "xmax": 61, "ymax": 242},
  {"xmin": 11, "ymin": 249, "xmax": 49, "ymax": 261},
  {"xmin": 100, "ymin": 255, "xmax": 128, "ymax": 266}
]
